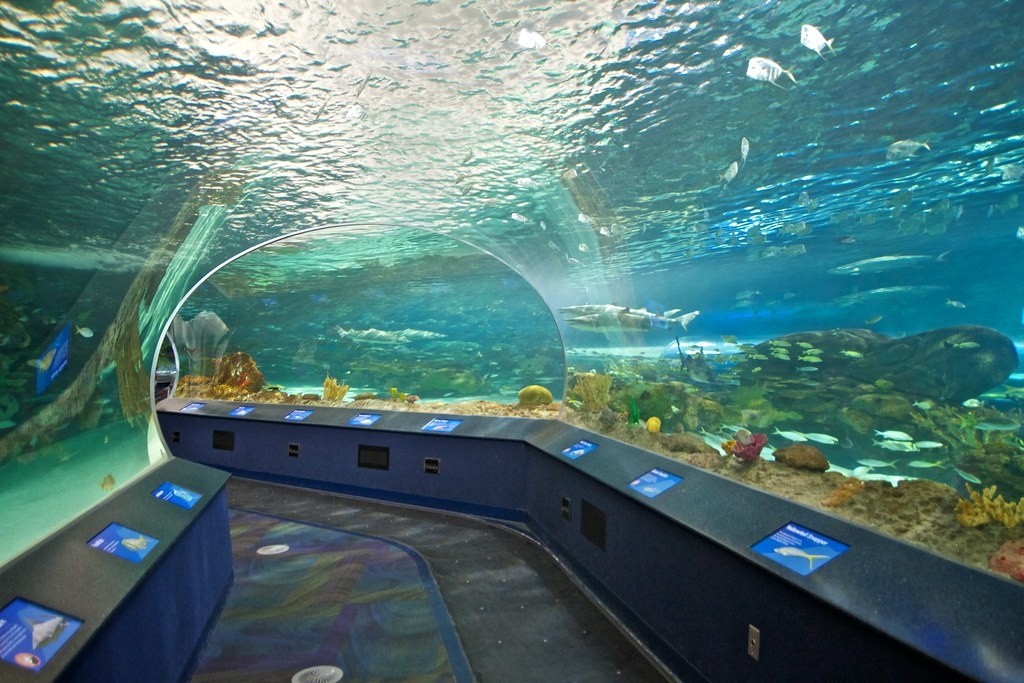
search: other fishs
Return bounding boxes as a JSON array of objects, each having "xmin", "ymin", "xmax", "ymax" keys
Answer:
[
  {"xmin": 0, "ymin": 283, "xmax": 116, "ymax": 492},
  {"xmin": 455, "ymin": 135, "xmax": 1023, "ymax": 487},
  {"xmin": 746, "ymin": 24, "xmax": 836, "ymax": 91}
]
